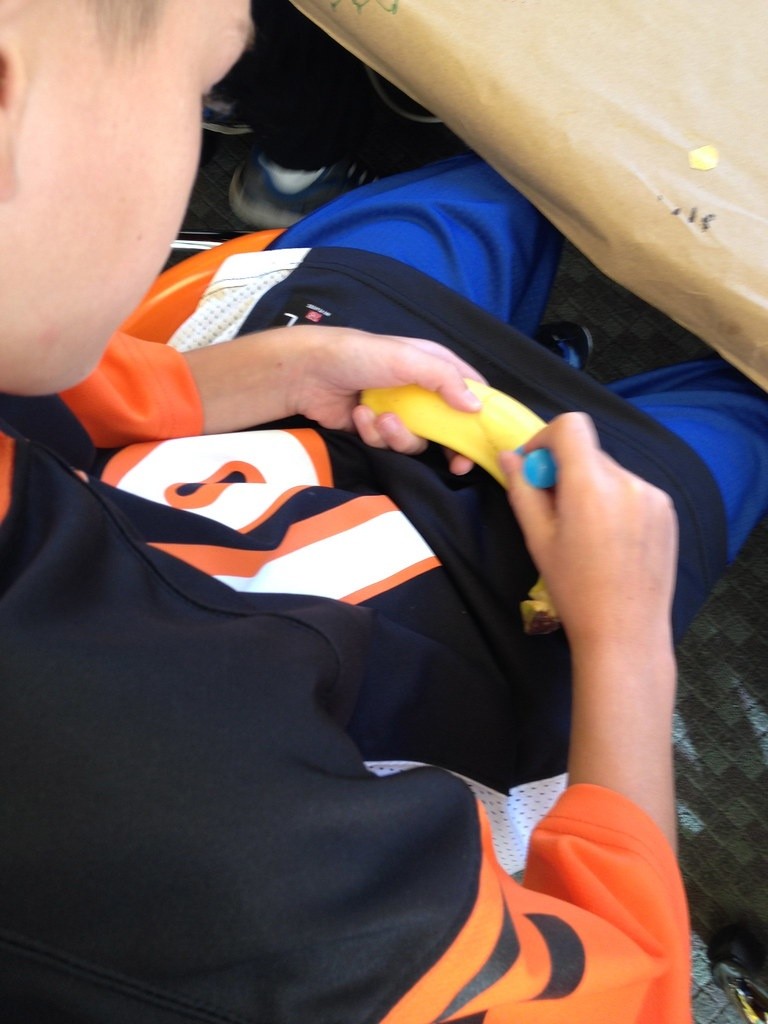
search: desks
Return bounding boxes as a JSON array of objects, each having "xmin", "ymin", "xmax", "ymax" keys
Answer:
[{"xmin": 285, "ymin": 0, "xmax": 767, "ymax": 407}]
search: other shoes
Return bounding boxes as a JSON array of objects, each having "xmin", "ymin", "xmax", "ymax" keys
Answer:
[
  {"xmin": 538, "ymin": 320, "xmax": 592, "ymax": 369},
  {"xmin": 204, "ymin": 86, "xmax": 348, "ymax": 229}
]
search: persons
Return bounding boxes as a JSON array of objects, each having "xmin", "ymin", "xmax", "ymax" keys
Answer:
[{"xmin": 0, "ymin": 0, "xmax": 768, "ymax": 1023}]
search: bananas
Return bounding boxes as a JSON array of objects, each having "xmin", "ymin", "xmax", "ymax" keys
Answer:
[{"xmin": 357, "ymin": 377, "xmax": 564, "ymax": 636}]
